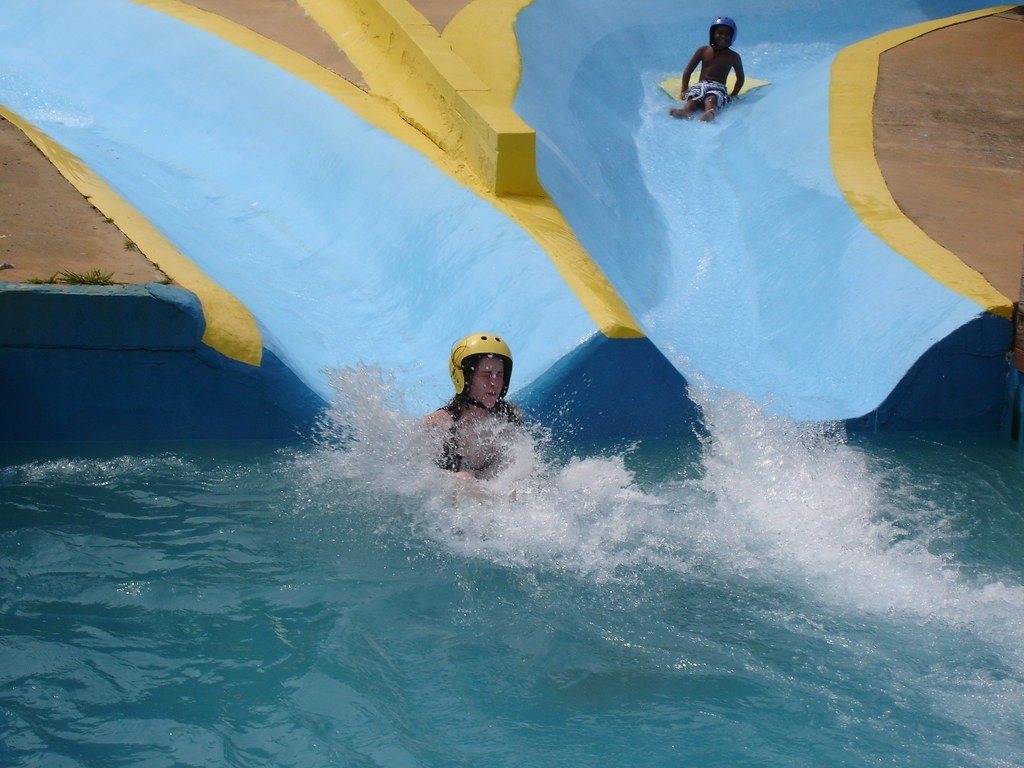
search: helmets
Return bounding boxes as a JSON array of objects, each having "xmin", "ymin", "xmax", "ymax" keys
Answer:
[
  {"xmin": 709, "ymin": 17, "xmax": 737, "ymax": 47},
  {"xmin": 450, "ymin": 332, "xmax": 512, "ymax": 399}
]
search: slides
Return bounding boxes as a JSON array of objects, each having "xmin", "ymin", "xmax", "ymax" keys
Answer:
[
  {"xmin": 0, "ymin": 2, "xmax": 611, "ymax": 442},
  {"xmin": 443, "ymin": 0, "xmax": 1023, "ymax": 422}
]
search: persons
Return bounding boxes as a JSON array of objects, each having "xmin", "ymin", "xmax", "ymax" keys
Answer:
[
  {"xmin": 414, "ymin": 332, "xmax": 528, "ymax": 497},
  {"xmin": 667, "ymin": 17, "xmax": 744, "ymax": 120}
]
